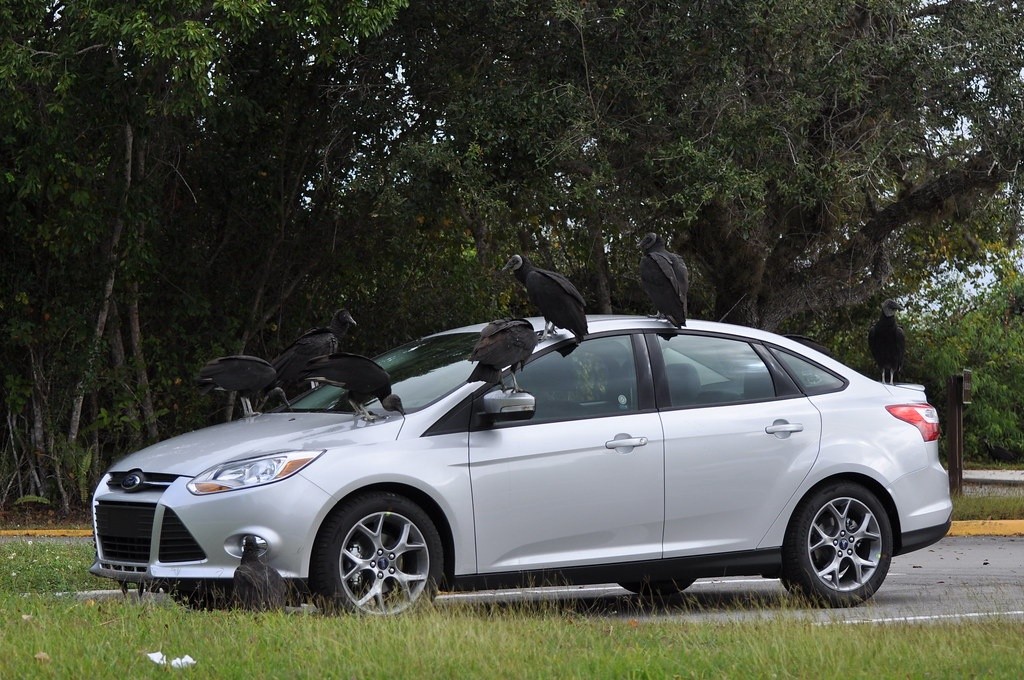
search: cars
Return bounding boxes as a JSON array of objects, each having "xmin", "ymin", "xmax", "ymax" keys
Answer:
[{"xmin": 88, "ymin": 314, "xmax": 955, "ymax": 626}]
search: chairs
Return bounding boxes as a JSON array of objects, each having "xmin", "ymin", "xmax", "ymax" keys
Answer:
[{"xmin": 520, "ymin": 355, "xmax": 776, "ymax": 417}]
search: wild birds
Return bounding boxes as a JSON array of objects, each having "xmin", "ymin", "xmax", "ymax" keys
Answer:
[
  {"xmin": 637, "ymin": 232, "xmax": 690, "ymax": 327},
  {"xmin": 193, "ymin": 355, "xmax": 291, "ymax": 415},
  {"xmin": 270, "ymin": 309, "xmax": 359, "ymax": 390},
  {"xmin": 298, "ymin": 352, "xmax": 404, "ymax": 423},
  {"xmin": 869, "ymin": 299, "xmax": 906, "ymax": 385},
  {"xmin": 502, "ymin": 253, "xmax": 591, "ymax": 346},
  {"xmin": 466, "ymin": 317, "xmax": 538, "ymax": 393}
]
